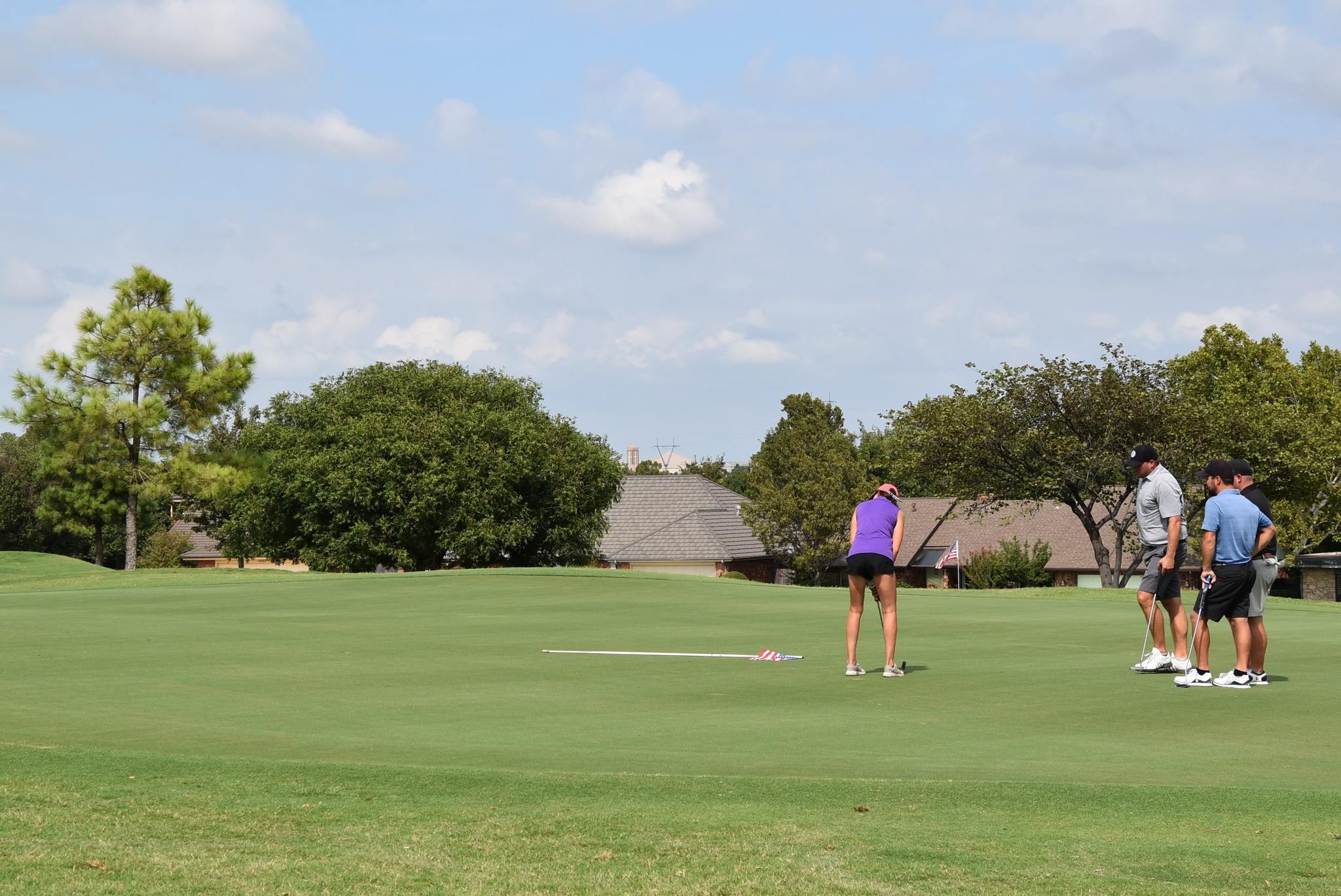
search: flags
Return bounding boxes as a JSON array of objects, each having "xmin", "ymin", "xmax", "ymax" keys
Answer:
[
  {"xmin": 747, "ymin": 649, "xmax": 803, "ymax": 662},
  {"xmin": 935, "ymin": 541, "xmax": 957, "ymax": 569}
]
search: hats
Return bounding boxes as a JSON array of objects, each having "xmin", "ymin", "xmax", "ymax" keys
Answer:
[
  {"xmin": 1125, "ymin": 445, "xmax": 1158, "ymax": 467},
  {"xmin": 1196, "ymin": 460, "xmax": 1234, "ymax": 479},
  {"xmin": 868, "ymin": 484, "xmax": 903, "ymax": 503},
  {"xmin": 1229, "ymin": 460, "xmax": 1253, "ymax": 476}
]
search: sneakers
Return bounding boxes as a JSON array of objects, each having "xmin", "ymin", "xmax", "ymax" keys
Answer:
[
  {"xmin": 883, "ymin": 663, "xmax": 904, "ymax": 677},
  {"xmin": 1174, "ymin": 669, "xmax": 1213, "ymax": 686},
  {"xmin": 1219, "ymin": 668, "xmax": 1269, "ymax": 685},
  {"xmin": 1134, "ymin": 647, "xmax": 1173, "ymax": 672},
  {"xmin": 1153, "ymin": 652, "xmax": 1192, "ymax": 673},
  {"xmin": 1213, "ymin": 671, "xmax": 1251, "ymax": 688},
  {"xmin": 845, "ymin": 663, "xmax": 866, "ymax": 676}
]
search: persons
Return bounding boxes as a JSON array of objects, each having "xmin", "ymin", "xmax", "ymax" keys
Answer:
[
  {"xmin": 1173, "ymin": 460, "xmax": 1277, "ymax": 690},
  {"xmin": 845, "ymin": 483, "xmax": 906, "ymax": 678},
  {"xmin": 1218, "ymin": 458, "xmax": 1282, "ymax": 685},
  {"xmin": 1130, "ymin": 444, "xmax": 1193, "ymax": 672}
]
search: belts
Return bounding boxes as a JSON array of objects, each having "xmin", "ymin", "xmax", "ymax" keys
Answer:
[
  {"xmin": 1251, "ymin": 554, "xmax": 1275, "ymax": 560},
  {"xmin": 1212, "ymin": 563, "xmax": 1233, "ymax": 566},
  {"xmin": 1145, "ymin": 539, "xmax": 1186, "ymax": 549}
]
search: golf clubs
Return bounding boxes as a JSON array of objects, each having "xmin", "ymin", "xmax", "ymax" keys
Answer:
[
  {"xmin": 1175, "ymin": 574, "xmax": 1211, "ymax": 688},
  {"xmin": 1129, "ymin": 565, "xmax": 1163, "ymax": 671},
  {"xmin": 875, "ymin": 596, "xmax": 906, "ymax": 673}
]
